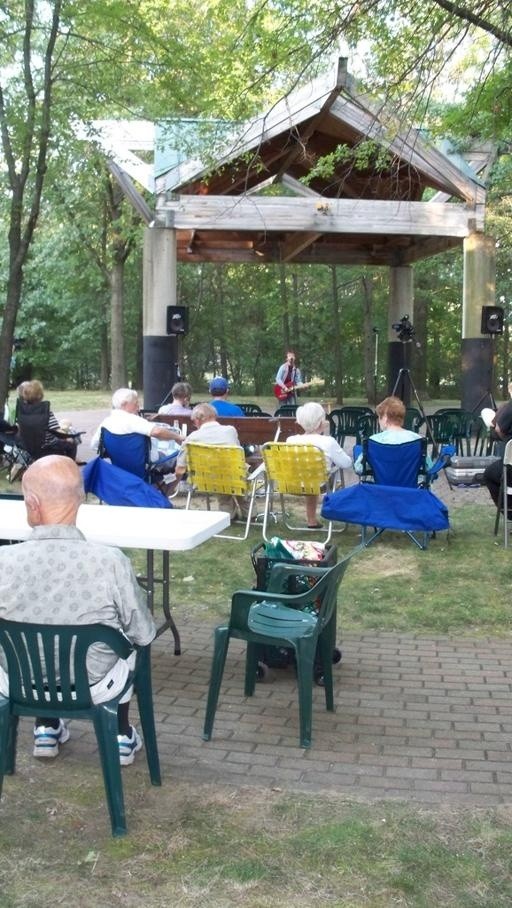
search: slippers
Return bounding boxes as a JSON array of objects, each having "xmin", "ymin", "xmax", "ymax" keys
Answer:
[{"xmin": 309, "ymin": 520, "xmax": 323, "ymax": 528}]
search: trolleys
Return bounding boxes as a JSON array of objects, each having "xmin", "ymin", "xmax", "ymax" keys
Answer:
[{"xmin": 248, "ymin": 536, "xmax": 342, "ymax": 686}]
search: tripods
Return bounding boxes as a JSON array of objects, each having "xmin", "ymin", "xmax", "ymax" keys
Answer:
[
  {"xmin": 159, "ymin": 334, "xmax": 193, "ymax": 409},
  {"xmin": 390, "ymin": 339, "xmax": 453, "ymax": 491},
  {"xmin": 471, "ymin": 334, "xmax": 497, "ymax": 415}
]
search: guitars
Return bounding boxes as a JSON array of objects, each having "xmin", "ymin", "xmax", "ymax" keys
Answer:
[{"xmin": 274, "ymin": 380, "xmax": 324, "ymax": 400}]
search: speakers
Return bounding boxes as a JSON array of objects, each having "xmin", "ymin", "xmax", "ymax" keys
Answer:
[
  {"xmin": 460, "ymin": 338, "xmax": 495, "ymax": 416},
  {"xmin": 167, "ymin": 305, "xmax": 189, "ymax": 334},
  {"xmin": 481, "ymin": 306, "xmax": 503, "ymax": 333},
  {"xmin": 389, "ymin": 342, "xmax": 411, "ymax": 408},
  {"xmin": 143, "ymin": 336, "xmax": 178, "ymax": 412}
]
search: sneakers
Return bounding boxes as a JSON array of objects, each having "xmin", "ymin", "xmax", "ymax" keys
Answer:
[
  {"xmin": 33, "ymin": 718, "xmax": 69, "ymax": 757},
  {"xmin": 117, "ymin": 725, "xmax": 142, "ymax": 766}
]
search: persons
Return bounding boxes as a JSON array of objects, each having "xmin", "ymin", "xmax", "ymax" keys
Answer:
[
  {"xmin": 91, "ymin": 387, "xmax": 186, "ymax": 499},
  {"xmin": 286, "ymin": 400, "xmax": 354, "ymax": 529},
  {"xmin": 207, "ymin": 376, "xmax": 255, "ymax": 455},
  {"xmin": 172, "ymin": 402, "xmax": 256, "ymax": 520},
  {"xmin": 157, "ymin": 381, "xmax": 194, "ymax": 417},
  {"xmin": 482, "ymin": 380, "xmax": 512, "ymax": 536},
  {"xmin": 354, "ymin": 396, "xmax": 435, "ymax": 488},
  {"xmin": 1, "ymin": 378, "xmax": 87, "ymax": 482},
  {"xmin": 0, "ymin": 455, "xmax": 156, "ymax": 766},
  {"xmin": 274, "ymin": 348, "xmax": 310, "ymax": 415}
]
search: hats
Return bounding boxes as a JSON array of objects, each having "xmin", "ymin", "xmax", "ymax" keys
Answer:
[{"xmin": 210, "ymin": 377, "xmax": 231, "ymax": 391}]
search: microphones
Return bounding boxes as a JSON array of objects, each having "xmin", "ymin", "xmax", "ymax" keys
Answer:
[
  {"xmin": 289, "ymin": 355, "xmax": 295, "ymax": 367},
  {"xmin": 372, "ymin": 326, "xmax": 380, "ymax": 333}
]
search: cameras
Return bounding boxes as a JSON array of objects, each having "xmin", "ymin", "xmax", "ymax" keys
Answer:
[{"xmin": 392, "ymin": 314, "xmax": 416, "ymax": 338}]
